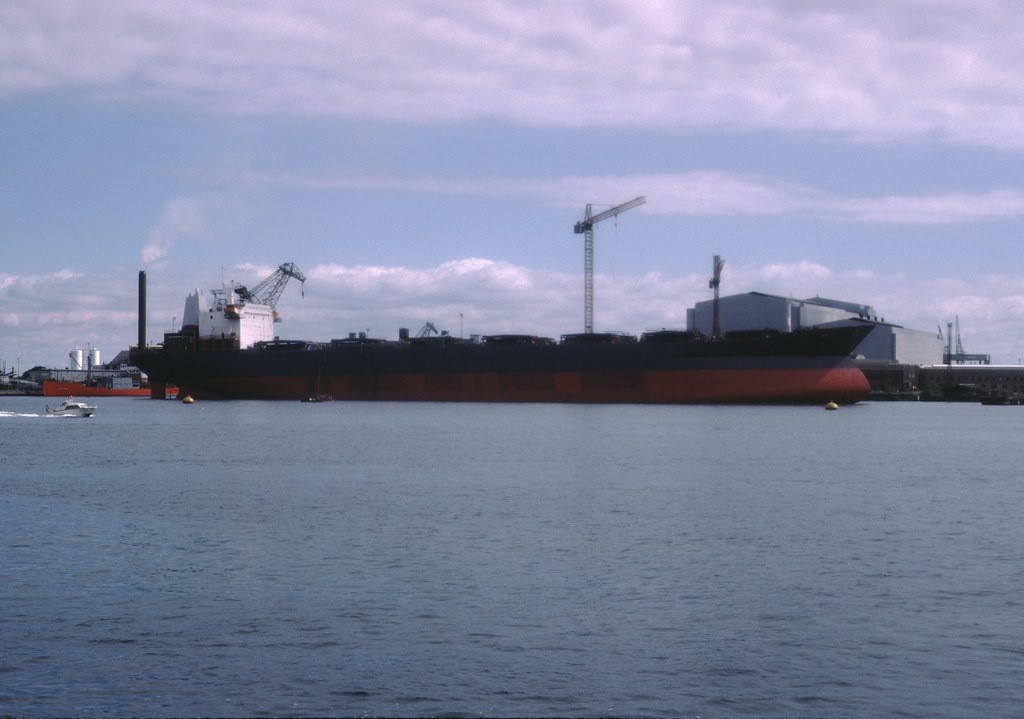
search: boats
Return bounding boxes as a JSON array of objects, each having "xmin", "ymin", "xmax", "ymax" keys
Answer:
[
  {"xmin": 42, "ymin": 381, "xmax": 180, "ymax": 397},
  {"xmin": 301, "ymin": 394, "xmax": 334, "ymax": 403},
  {"xmin": 183, "ymin": 395, "xmax": 193, "ymax": 403},
  {"xmin": 44, "ymin": 401, "xmax": 97, "ymax": 418}
]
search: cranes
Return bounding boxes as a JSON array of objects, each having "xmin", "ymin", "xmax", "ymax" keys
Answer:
[
  {"xmin": 709, "ymin": 255, "xmax": 724, "ymax": 342},
  {"xmin": 574, "ymin": 195, "xmax": 647, "ymax": 334}
]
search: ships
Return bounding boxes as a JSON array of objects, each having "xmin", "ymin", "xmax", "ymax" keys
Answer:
[{"xmin": 141, "ymin": 283, "xmax": 876, "ymax": 404}]
